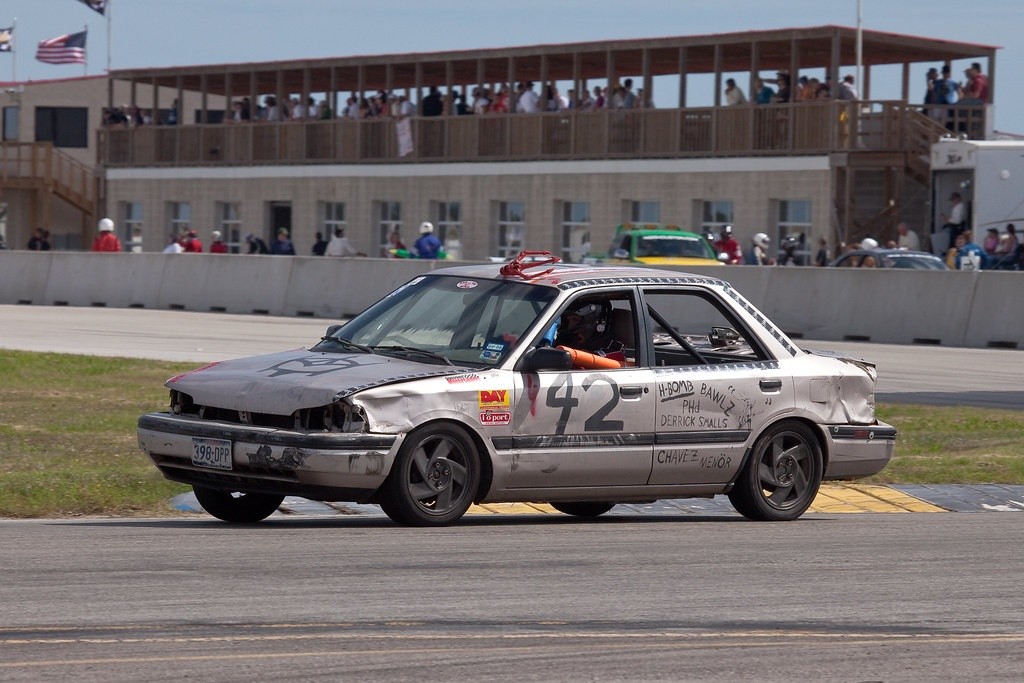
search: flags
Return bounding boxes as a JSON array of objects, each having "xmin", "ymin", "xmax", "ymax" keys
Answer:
[
  {"xmin": 0, "ymin": 26, "xmax": 15, "ymax": 52},
  {"xmin": 78, "ymin": 0, "xmax": 107, "ymax": 18},
  {"xmin": 34, "ymin": 31, "xmax": 87, "ymax": 65}
]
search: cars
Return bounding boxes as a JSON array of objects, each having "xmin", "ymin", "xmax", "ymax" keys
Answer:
[
  {"xmin": 828, "ymin": 249, "xmax": 950, "ymax": 269},
  {"xmin": 136, "ymin": 262, "xmax": 898, "ymax": 521}
]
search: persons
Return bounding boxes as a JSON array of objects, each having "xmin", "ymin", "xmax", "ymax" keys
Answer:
[
  {"xmin": 246, "ymin": 228, "xmax": 296, "ymax": 256},
  {"xmin": 383, "ymin": 230, "xmax": 405, "ymax": 258},
  {"xmin": 311, "ymin": 229, "xmax": 367, "ymax": 257},
  {"xmin": 406, "ymin": 221, "xmax": 441, "ymax": 259},
  {"xmin": 90, "ymin": 217, "xmax": 122, "ymax": 252},
  {"xmin": 27, "ymin": 227, "xmax": 50, "ymax": 250},
  {"xmin": 162, "ymin": 227, "xmax": 229, "ymax": 253},
  {"xmin": 503, "ymin": 298, "xmax": 628, "ymax": 369},
  {"xmin": 724, "ymin": 71, "xmax": 870, "ymax": 150},
  {"xmin": 921, "ymin": 60, "xmax": 989, "ymax": 133},
  {"xmin": 104, "ymin": 78, "xmax": 656, "ymax": 161},
  {"xmin": 699, "ymin": 191, "xmax": 1020, "ymax": 268}
]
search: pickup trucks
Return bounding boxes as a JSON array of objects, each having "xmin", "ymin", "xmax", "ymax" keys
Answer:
[{"xmin": 583, "ymin": 222, "xmax": 729, "ymax": 266}]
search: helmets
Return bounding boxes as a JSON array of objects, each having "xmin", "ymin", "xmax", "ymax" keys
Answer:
[
  {"xmin": 279, "ymin": 227, "xmax": 288, "ymax": 235},
  {"xmin": 419, "ymin": 221, "xmax": 434, "ymax": 234},
  {"xmin": 702, "ymin": 232, "xmax": 714, "ymax": 242},
  {"xmin": 97, "ymin": 217, "xmax": 114, "ymax": 232},
  {"xmin": 556, "ymin": 295, "xmax": 613, "ymax": 348},
  {"xmin": 752, "ymin": 233, "xmax": 770, "ymax": 250},
  {"xmin": 721, "ymin": 225, "xmax": 731, "ymax": 235},
  {"xmin": 211, "ymin": 231, "xmax": 223, "ymax": 243}
]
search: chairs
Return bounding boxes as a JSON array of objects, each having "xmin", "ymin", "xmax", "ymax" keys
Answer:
[{"xmin": 610, "ymin": 307, "xmax": 637, "ymax": 349}]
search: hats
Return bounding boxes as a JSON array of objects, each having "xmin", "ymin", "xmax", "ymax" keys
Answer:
[
  {"xmin": 187, "ymin": 230, "xmax": 197, "ymax": 235},
  {"xmin": 855, "ymin": 238, "xmax": 879, "ymax": 252},
  {"xmin": 948, "ymin": 192, "xmax": 960, "ymax": 201},
  {"xmin": 987, "ymin": 228, "xmax": 998, "ymax": 235}
]
